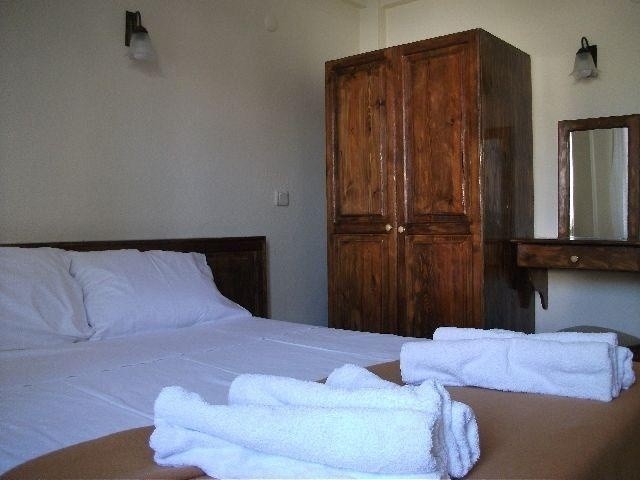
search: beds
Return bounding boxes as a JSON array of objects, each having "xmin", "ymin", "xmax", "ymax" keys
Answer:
[{"xmin": 1, "ymin": 236, "xmax": 640, "ymax": 480}]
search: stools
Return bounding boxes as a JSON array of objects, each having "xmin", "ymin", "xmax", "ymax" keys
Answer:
[{"xmin": 558, "ymin": 325, "xmax": 640, "ymax": 361}]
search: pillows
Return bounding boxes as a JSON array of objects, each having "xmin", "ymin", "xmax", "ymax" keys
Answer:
[
  {"xmin": 68, "ymin": 249, "xmax": 251, "ymax": 342},
  {"xmin": 0, "ymin": 247, "xmax": 96, "ymax": 352}
]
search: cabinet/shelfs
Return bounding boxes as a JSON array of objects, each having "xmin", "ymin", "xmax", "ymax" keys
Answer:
[{"xmin": 325, "ymin": 28, "xmax": 535, "ymax": 336}]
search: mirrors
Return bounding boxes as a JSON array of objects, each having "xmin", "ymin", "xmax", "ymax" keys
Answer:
[{"xmin": 558, "ymin": 114, "xmax": 640, "ymax": 241}]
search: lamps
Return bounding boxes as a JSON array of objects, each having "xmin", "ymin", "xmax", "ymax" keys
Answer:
[
  {"xmin": 569, "ymin": 36, "xmax": 603, "ymax": 84},
  {"xmin": 124, "ymin": 9, "xmax": 159, "ymax": 62}
]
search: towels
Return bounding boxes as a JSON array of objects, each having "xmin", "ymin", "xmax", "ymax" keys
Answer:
[
  {"xmin": 399, "ymin": 327, "xmax": 636, "ymax": 404},
  {"xmin": 147, "ymin": 364, "xmax": 481, "ymax": 478}
]
